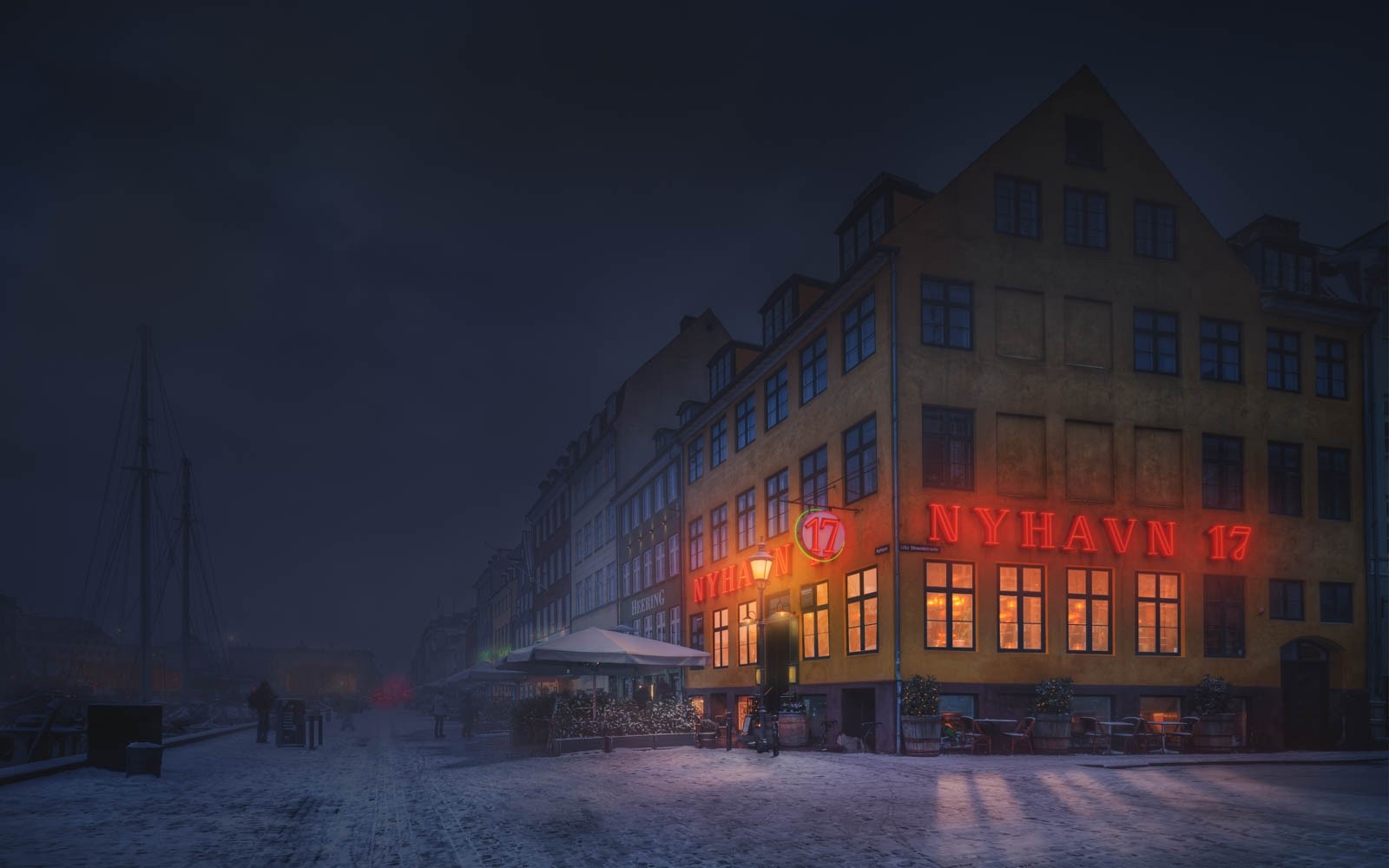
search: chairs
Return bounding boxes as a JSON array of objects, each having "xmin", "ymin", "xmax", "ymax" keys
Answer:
[
  {"xmin": 1167, "ymin": 716, "xmax": 1201, "ymax": 753},
  {"xmin": 961, "ymin": 715, "xmax": 995, "ymax": 756},
  {"xmin": 1080, "ymin": 714, "xmax": 1111, "ymax": 754},
  {"xmin": 1116, "ymin": 716, "xmax": 1145, "ymax": 753},
  {"xmin": 1002, "ymin": 717, "xmax": 1037, "ymax": 754}
]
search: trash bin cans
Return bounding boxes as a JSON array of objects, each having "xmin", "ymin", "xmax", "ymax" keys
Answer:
[{"xmin": 277, "ymin": 697, "xmax": 305, "ymax": 746}]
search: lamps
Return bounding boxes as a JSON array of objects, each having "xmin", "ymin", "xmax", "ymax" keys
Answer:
[
  {"xmin": 647, "ymin": 530, "xmax": 653, "ymax": 535},
  {"xmin": 660, "ymin": 521, "xmax": 666, "ymax": 526},
  {"xmin": 626, "ymin": 544, "xmax": 631, "ymax": 549},
  {"xmin": 635, "ymin": 536, "xmax": 642, "ymax": 543},
  {"xmin": 742, "ymin": 611, "xmax": 756, "ymax": 622}
]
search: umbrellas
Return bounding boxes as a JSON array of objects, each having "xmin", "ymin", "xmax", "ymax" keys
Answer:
[
  {"xmin": 413, "ymin": 646, "xmax": 526, "ymax": 702},
  {"xmin": 507, "ymin": 626, "xmax": 711, "ymax": 736}
]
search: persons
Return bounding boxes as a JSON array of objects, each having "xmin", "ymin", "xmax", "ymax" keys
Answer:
[
  {"xmin": 256, "ymin": 680, "xmax": 274, "ymax": 743},
  {"xmin": 633, "ymin": 684, "xmax": 652, "ymax": 701},
  {"xmin": 657, "ymin": 677, "xmax": 668, "ymax": 695},
  {"xmin": 341, "ymin": 706, "xmax": 355, "ymax": 731},
  {"xmin": 430, "ymin": 689, "xmax": 450, "ymax": 738}
]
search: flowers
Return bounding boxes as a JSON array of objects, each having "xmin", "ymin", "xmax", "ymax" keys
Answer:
[
  {"xmin": 780, "ymin": 703, "xmax": 807, "ymax": 714},
  {"xmin": 700, "ymin": 719, "xmax": 717, "ymax": 733},
  {"xmin": 1031, "ymin": 677, "xmax": 1075, "ymax": 715},
  {"xmin": 898, "ymin": 674, "xmax": 942, "ymax": 716},
  {"xmin": 1195, "ymin": 674, "xmax": 1232, "ymax": 713}
]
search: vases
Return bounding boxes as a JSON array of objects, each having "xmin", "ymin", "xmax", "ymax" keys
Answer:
[
  {"xmin": 779, "ymin": 711, "xmax": 805, "ymax": 747},
  {"xmin": 1190, "ymin": 712, "xmax": 1246, "ymax": 753},
  {"xmin": 900, "ymin": 710, "xmax": 942, "ymax": 755},
  {"xmin": 697, "ymin": 731, "xmax": 716, "ymax": 748},
  {"xmin": 1029, "ymin": 710, "xmax": 1071, "ymax": 756}
]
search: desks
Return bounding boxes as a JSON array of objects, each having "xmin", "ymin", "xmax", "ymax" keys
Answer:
[
  {"xmin": 1101, "ymin": 722, "xmax": 1133, "ymax": 753},
  {"xmin": 973, "ymin": 717, "xmax": 1016, "ymax": 755},
  {"xmin": 1144, "ymin": 721, "xmax": 1187, "ymax": 754}
]
